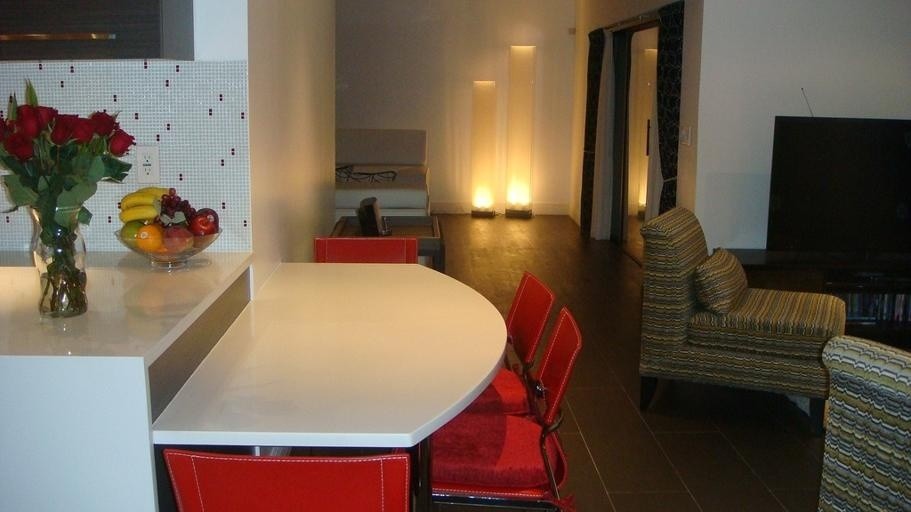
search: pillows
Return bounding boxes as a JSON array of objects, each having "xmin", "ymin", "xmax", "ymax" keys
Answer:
[{"xmin": 692, "ymin": 243, "xmax": 752, "ymax": 317}]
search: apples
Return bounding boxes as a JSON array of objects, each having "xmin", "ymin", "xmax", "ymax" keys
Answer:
[
  {"xmin": 161, "ymin": 226, "xmax": 194, "ymax": 253},
  {"xmin": 190, "ymin": 216, "xmax": 216, "ymax": 235},
  {"xmin": 197, "ymin": 208, "xmax": 219, "ymax": 231}
]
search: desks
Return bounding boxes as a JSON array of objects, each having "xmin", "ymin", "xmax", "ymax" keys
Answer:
[{"xmin": 151, "ymin": 259, "xmax": 514, "ymax": 511}]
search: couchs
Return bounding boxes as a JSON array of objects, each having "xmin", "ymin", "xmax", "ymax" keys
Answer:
[{"xmin": 335, "ymin": 124, "xmax": 436, "ymax": 216}]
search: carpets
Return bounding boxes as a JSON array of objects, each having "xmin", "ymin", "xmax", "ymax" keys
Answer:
[{"xmin": 789, "ymin": 389, "xmax": 831, "ymax": 432}]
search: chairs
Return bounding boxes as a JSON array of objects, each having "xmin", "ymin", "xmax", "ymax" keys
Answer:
[
  {"xmin": 633, "ymin": 202, "xmax": 854, "ymax": 440},
  {"xmin": 419, "ymin": 301, "xmax": 584, "ymax": 511},
  {"xmin": 465, "ymin": 269, "xmax": 558, "ymax": 420},
  {"xmin": 816, "ymin": 333, "xmax": 911, "ymax": 511},
  {"xmin": 307, "ymin": 232, "xmax": 421, "ymax": 264},
  {"xmin": 160, "ymin": 442, "xmax": 414, "ymax": 512}
]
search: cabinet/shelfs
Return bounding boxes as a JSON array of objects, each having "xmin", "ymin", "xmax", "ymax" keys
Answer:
[{"xmin": 713, "ymin": 245, "xmax": 911, "ymax": 351}]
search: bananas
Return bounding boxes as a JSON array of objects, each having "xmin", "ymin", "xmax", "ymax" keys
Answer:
[{"xmin": 119, "ymin": 186, "xmax": 171, "ymax": 223}]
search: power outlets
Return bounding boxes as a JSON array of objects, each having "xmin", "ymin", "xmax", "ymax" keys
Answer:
[{"xmin": 136, "ymin": 145, "xmax": 162, "ymax": 184}]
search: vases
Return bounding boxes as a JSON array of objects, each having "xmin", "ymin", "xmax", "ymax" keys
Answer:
[{"xmin": 26, "ymin": 201, "xmax": 94, "ymax": 317}]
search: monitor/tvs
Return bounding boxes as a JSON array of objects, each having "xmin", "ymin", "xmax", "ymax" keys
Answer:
[{"xmin": 766, "ymin": 115, "xmax": 911, "ymax": 252}]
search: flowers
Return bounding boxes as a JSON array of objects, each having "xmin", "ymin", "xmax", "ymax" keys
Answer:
[{"xmin": 0, "ymin": 74, "xmax": 138, "ymax": 315}]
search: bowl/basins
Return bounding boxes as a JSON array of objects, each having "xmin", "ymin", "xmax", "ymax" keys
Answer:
[{"xmin": 114, "ymin": 228, "xmax": 224, "ymax": 267}]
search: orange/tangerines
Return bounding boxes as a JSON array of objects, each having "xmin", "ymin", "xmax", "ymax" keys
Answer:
[{"xmin": 119, "ymin": 222, "xmax": 145, "ymax": 248}]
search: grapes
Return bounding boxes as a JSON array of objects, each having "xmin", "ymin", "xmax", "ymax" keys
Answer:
[{"xmin": 154, "ymin": 188, "xmax": 195, "ymax": 225}]
